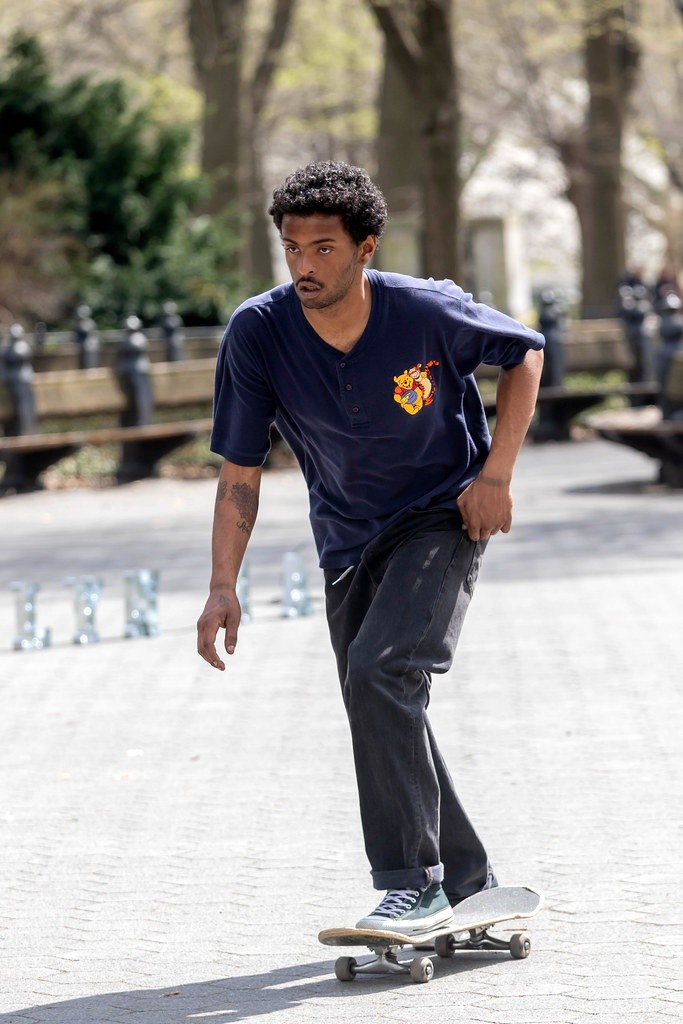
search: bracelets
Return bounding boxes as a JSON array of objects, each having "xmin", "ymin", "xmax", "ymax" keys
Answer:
[{"xmin": 478, "ymin": 472, "xmax": 508, "ymax": 487}]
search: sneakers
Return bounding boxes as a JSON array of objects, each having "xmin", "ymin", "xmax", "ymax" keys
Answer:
[
  {"xmin": 450, "ymin": 860, "xmax": 500, "ymax": 909},
  {"xmin": 355, "ymin": 881, "xmax": 454, "ymax": 936}
]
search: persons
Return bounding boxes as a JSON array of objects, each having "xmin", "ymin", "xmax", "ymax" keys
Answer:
[{"xmin": 195, "ymin": 160, "xmax": 546, "ymax": 932}]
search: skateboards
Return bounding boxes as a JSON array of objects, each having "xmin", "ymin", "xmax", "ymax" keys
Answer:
[{"xmin": 315, "ymin": 885, "xmax": 546, "ymax": 984}]
